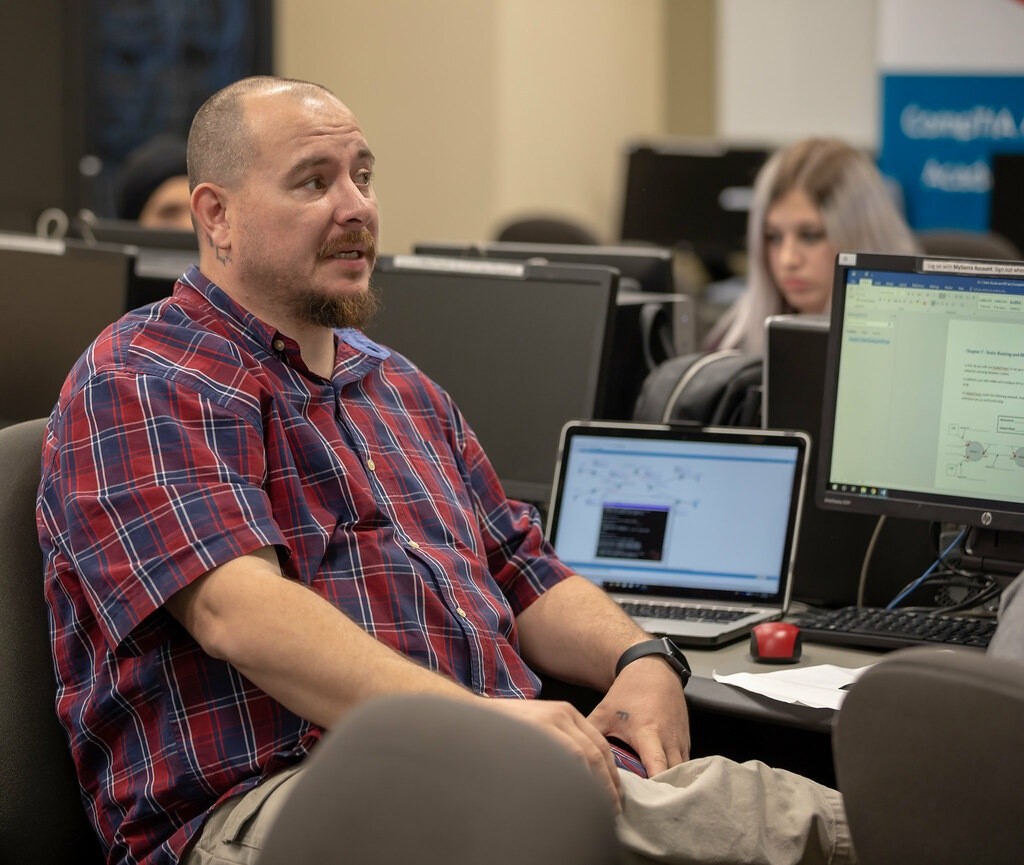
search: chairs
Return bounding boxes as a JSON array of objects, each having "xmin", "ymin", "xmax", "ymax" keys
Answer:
[
  {"xmin": 0, "ymin": 417, "xmax": 100, "ymax": 865},
  {"xmin": 834, "ymin": 640, "xmax": 1024, "ymax": 865}
]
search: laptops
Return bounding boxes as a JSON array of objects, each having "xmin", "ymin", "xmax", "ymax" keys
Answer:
[{"xmin": 547, "ymin": 419, "xmax": 812, "ymax": 647}]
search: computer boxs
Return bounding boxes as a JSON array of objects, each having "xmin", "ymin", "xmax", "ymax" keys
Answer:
[{"xmin": 765, "ymin": 313, "xmax": 944, "ymax": 613}]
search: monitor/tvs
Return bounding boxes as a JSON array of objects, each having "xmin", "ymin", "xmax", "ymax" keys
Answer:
[
  {"xmin": 349, "ymin": 238, "xmax": 691, "ymax": 541},
  {"xmin": 0, "ymin": 224, "xmax": 200, "ymax": 434},
  {"xmin": 815, "ymin": 250, "xmax": 1024, "ymax": 619}
]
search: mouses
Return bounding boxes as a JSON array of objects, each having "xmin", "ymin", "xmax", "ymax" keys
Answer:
[{"xmin": 749, "ymin": 621, "xmax": 805, "ymax": 663}]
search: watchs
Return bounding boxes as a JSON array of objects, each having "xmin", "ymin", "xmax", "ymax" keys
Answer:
[{"xmin": 615, "ymin": 636, "xmax": 691, "ymax": 689}]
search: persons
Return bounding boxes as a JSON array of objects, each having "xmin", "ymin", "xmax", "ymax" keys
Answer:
[
  {"xmin": 116, "ymin": 132, "xmax": 196, "ymax": 235},
  {"xmin": 708, "ymin": 135, "xmax": 931, "ymax": 353},
  {"xmin": 33, "ymin": 75, "xmax": 849, "ymax": 865}
]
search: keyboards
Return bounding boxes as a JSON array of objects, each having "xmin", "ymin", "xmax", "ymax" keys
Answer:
[{"xmin": 793, "ymin": 606, "xmax": 998, "ymax": 651}]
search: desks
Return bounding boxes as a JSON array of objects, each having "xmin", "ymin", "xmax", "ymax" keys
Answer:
[{"xmin": 682, "ymin": 604, "xmax": 878, "ymax": 727}]
